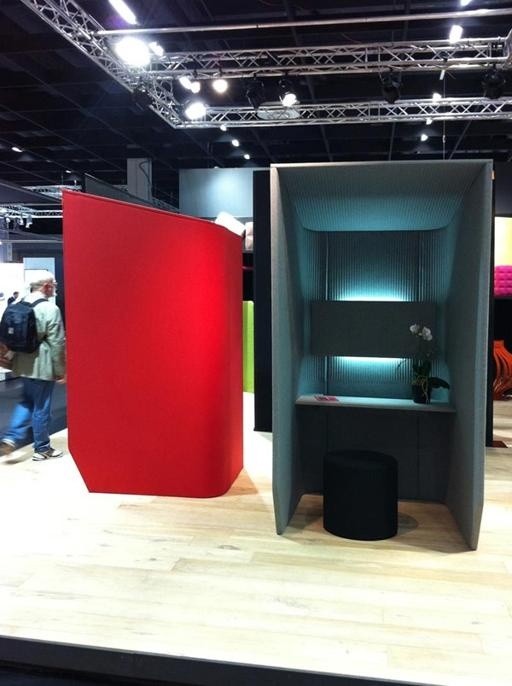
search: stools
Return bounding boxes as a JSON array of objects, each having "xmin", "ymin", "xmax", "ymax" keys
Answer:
[{"xmin": 322, "ymin": 449, "xmax": 400, "ymax": 539}]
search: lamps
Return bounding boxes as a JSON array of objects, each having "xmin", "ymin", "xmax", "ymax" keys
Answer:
[
  {"xmin": 378, "ymin": 71, "xmax": 401, "ymax": 103},
  {"xmin": 246, "ymin": 70, "xmax": 297, "ymax": 109}
]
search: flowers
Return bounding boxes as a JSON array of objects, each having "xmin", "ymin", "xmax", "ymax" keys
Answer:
[{"xmin": 397, "ymin": 322, "xmax": 450, "ymax": 404}]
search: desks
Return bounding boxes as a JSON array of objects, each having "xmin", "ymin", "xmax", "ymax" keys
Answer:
[{"xmin": 273, "ymin": 394, "xmax": 488, "ymax": 551}]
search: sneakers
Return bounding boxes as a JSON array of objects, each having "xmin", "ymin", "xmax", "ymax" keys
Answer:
[
  {"xmin": 32, "ymin": 449, "xmax": 63, "ymax": 461},
  {"xmin": 0, "ymin": 439, "xmax": 16, "ymax": 454}
]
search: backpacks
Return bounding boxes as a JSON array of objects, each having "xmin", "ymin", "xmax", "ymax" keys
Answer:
[{"xmin": 0, "ymin": 294, "xmax": 48, "ymax": 354}]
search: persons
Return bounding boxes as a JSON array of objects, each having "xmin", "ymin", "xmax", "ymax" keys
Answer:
[
  {"xmin": 8, "ymin": 292, "xmax": 19, "ymax": 304},
  {"xmin": 0, "ymin": 271, "xmax": 65, "ymax": 461}
]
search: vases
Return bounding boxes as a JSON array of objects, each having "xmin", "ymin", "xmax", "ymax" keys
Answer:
[{"xmin": 412, "ymin": 384, "xmax": 432, "ymax": 403}]
